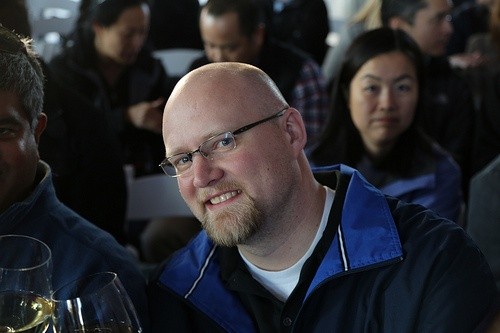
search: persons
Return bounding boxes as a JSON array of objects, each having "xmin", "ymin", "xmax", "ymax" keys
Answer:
[
  {"xmin": 163, "ymin": 0, "xmax": 328, "ymax": 156},
  {"xmin": 0, "ymin": 22, "xmax": 147, "ymax": 333},
  {"xmin": 140, "ymin": 61, "xmax": 500, "ymax": 333},
  {"xmin": 380, "ymin": 0, "xmax": 475, "ymax": 159},
  {"xmin": 307, "ymin": 27, "xmax": 467, "ymax": 231},
  {"xmin": 30, "ymin": 0, "xmax": 173, "ymax": 250}
]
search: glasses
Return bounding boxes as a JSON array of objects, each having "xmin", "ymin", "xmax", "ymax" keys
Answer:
[{"xmin": 158, "ymin": 107, "xmax": 288, "ymax": 177}]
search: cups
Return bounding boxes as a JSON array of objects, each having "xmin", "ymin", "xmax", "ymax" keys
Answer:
[
  {"xmin": 0, "ymin": 235, "xmax": 51, "ymax": 333},
  {"xmin": 50, "ymin": 272, "xmax": 143, "ymax": 333}
]
